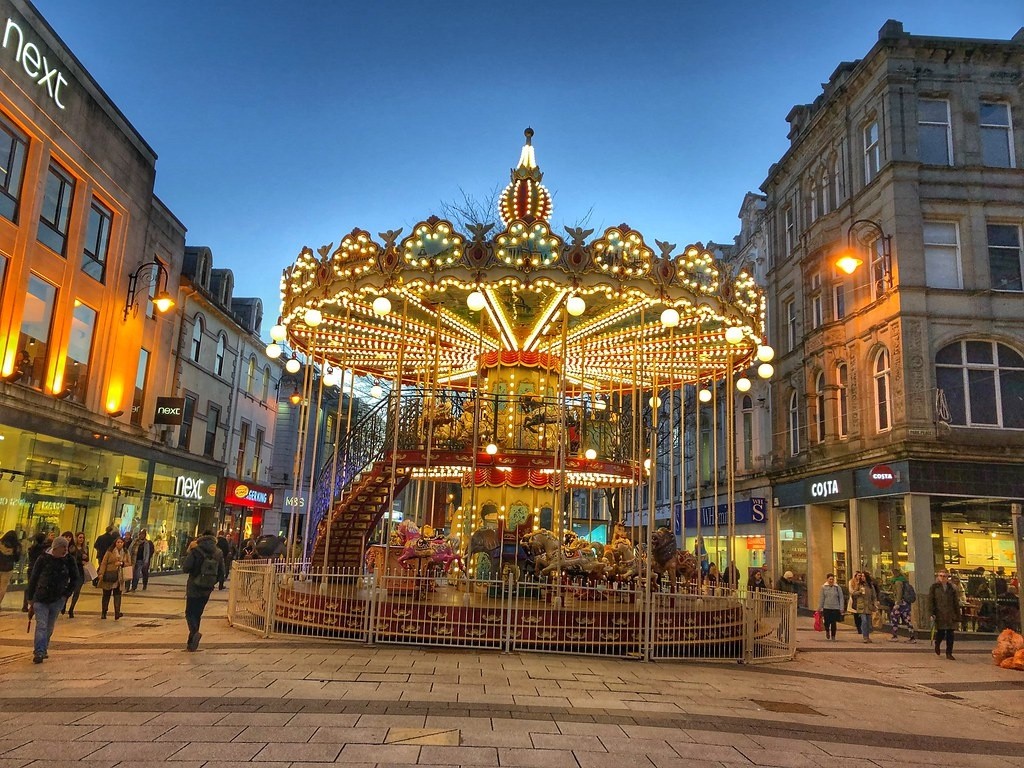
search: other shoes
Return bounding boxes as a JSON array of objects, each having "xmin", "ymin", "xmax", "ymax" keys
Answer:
[
  {"xmin": 858, "ymin": 630, "xmax": 862, "ymax": 634},
  {"xmin": 33, "ymin": 653, "xmax": 48, "ymax": 664},
  {"xmin": 68, "ymin": 609, "xmax": 74, "ymax": 618},
  {"xmin": 219, "ymin": 586, "xmax": 226, "ymax": 590},
  {"xmin": 61, "ymin": 604, "xmax": 66, "ymax": 615},
  {"xmin": 187, "ymin": 633, "xmax": 201, "ymax": 652},
  {"xmin": 905, "ymin": 638, "xmax": 918, "ymax": 644},
  {"xmin": 863, "ymin": 638, "xmax": 872, "ymax": 643},
  {"xmin": 946, "ymin": 653, "xmax": 955, "ymax": 661},
  {"xmin": 826, "ymin": 631, "xmax": 830, "ymax": 639},
  {"xmin": 101, "ymin": 611, "xmax": 106, "ymax": 619},
  {"xmin": 935, "ymin": 645, "xmax": 940, "ymax": 655},
  {"xmin": 888, "ymin": 637, "xmax": 899, "ymax": 642},
  {"xmin": 115, "ymin": 613, "xmax": 123, "ymax": 620}
]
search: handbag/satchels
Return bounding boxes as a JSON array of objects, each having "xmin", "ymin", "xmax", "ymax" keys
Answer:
[
  {"xmin": 871, "ymin": 609, "xmax": 883, "ymax": 630},
  {"xmin": 814, "ymin": 611, "xmax": 824, "ymax": 631},
  {"xmin": 83, "ymin": 561, "xmax": 98, "ymax": 581},
  {"xmin": 103, "ymin": 571, "xmax": 118, "ymax": 582},
  {"xmin": 122, "ymin": 566, "xmax": 133, "ymax": 581}
]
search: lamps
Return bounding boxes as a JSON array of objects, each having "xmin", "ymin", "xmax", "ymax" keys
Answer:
[
  {"xmin": 123, "ymin": 262, "xmax": 176, "ymax": 321},
  {"xmin": 953, "ymin": 522, "xmax": 966, "ymax": 558},
  {"xmin": 275, "ymin": 374, "xmax": 301, "ymax": 405},
  {"xmin": 835, "ymin": 219, "xmax": 893, "ymax": 288},
  {"xmin": 987, "ymin": 535, "xmax": 999, "ymax": 560}
]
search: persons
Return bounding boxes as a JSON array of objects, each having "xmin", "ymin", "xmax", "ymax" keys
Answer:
[
  {"xmin": 928, "ymin": 569, "xmax": 961, "ymax": 659},
  {"xmin": 888, "ymin": 569, "xmax": 918, "ymax": 644},
  {"xmin": 817, "ymin": 573, "xmax": 845, "ymax": 641},
  {"xmin": 747, "ymin": 569, "xmax": 766, "ymax": 633},
  {"xmin": 722, "ymin": 561, "xmax": 740, "ymax": 596},
  {"xmin": 26, "ymin": 536, "xmax": 78, "ymax": 664},
  {"xmin": 22, "ymin": 531, "xmax": 90, "ymax": 618},
  {"xmin": 98, "ymin": 537, "xmax": 131, "ymax": 620},
  {"xmin": 92, "ymin": 526, "xmax": 154, "ymax": 593},
  {"xmin": 708, "ymin": 562, "xmax": 723, "ymax": 596},
  {"xmin": 0, "ymin": 530, "xmax": 22, "ymax": 605},
  {"xmin": 156, "ymin": 519, "xmax": 177, "ymax": 571},
  {"xmin": 186, "ymin": 531, "xmax": 313, "ymax": 590},
  {"xmin": 847, "ymin": 570, "xmax": 880, "ymax": 644},
  {"xmin": 184, "ymin": 530, "xmax": 225, "ymax": 653},
  {"xmin": 774, "ymin": 570, "xmax": 794, "ymax": 643},
  {"xmin": 951, "ymin": 567, "xmax": 1020, "ymax": 632}
]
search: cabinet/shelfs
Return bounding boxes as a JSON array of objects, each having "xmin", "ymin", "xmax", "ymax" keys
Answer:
[{"xmin": 833, "ymin": 551, "xmax": 847, "ymax": 587}]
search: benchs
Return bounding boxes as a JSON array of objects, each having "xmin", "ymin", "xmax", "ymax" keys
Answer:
[{"xmin": 962, "ymin": 613, "xmax": 989, "ymax": 632}]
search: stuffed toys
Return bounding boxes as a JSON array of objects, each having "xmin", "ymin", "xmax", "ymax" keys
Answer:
[{"xmin": 991, "ymin": 629, "xmax": 1024, "ymax": 671}]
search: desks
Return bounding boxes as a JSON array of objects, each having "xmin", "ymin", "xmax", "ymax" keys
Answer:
[{"xmin": 960, "ymin": 605, "xmax": 978, "ymax": 631}]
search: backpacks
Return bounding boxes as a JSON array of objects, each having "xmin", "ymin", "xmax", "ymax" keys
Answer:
[
  {"xmin": 244, "ymin": 548, "xmax": 255, "ymax": 560},
  {"xmin": 193, "ymin": 546, "xmax": 222, "ymax": 588},
  {"xmin": 902, "ymin": 582, "xmax": 917, "ymax": 603}
]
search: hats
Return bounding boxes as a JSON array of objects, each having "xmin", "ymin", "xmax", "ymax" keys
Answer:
[
  {"xmin": 51, "ymin": 537, "xmax": 67, "ymax": 550},
  {"xmin": 784, "ymin": 571, "xmax": 793, "ymax": 579}
]
